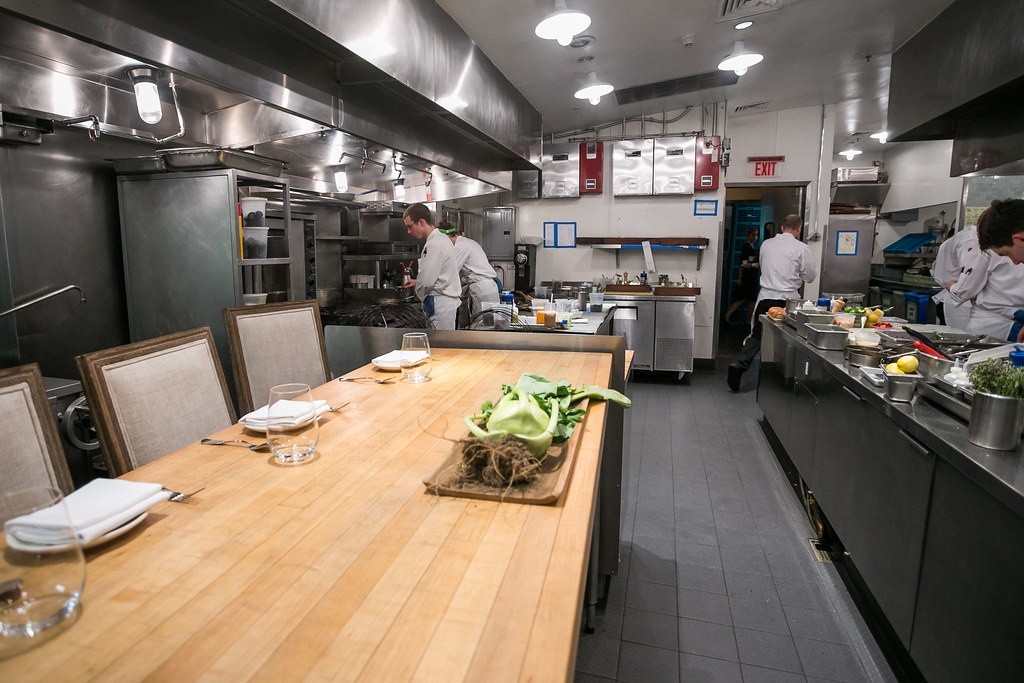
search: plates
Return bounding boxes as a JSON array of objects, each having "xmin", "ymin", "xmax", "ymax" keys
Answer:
[
  {"xmin": 245, "ymin": 415, "xmax": 323, "ymax": 433},
  {"xmin": 860, "ymin": 366, "xmax": 884, "ymax": 386},
  {"xmin": 5, "ymin": 510, "xmax": 163, "ymax": 553},
  {"xmin": 372, "ymin": 360, "xmax": 425, "ymax": 370}
]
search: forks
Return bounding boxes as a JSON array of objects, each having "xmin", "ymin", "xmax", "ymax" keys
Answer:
[
  {"xmin": 163, "ymin": 486, "xmax": 205, "ymax": 501},
  {"xmin": 339, "ymin": 377, "xmax": 393, "ymax": 384},
  {"xmin": 201, "ymin": 437, "xmax": 279, "ymax": 450}
]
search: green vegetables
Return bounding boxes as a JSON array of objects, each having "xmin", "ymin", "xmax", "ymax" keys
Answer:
[
  {"xmin": 464, "ymin": 373, "xmax": 632, "ymax": 457},
  {"xmin": 965, "ymin": 355, "xmax": 1024, "ymax": 398}
]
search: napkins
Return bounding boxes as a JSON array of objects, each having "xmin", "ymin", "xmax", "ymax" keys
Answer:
[
  {"xmin": 6, "ymin": 478, "xmax": 171, "ymax": 549},
  {"xmin": 239, "ymin": 400, "xmax": 332, "ymax": 431},
  {"xmin": 372, "ymin": 350, "xmax": 428, "ymax": 368}
]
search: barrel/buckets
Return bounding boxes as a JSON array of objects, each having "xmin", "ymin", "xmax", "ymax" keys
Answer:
[
  {"xmin": 243, "ymin": 227, "xmax": 269, "ymax": 259},
  {"xmin": 241, "ymin": 197, "xmax": 267, "ymax": 227}
]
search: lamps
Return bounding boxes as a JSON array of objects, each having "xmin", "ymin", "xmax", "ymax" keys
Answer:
[
  {"xmin": 573, "ymin": 71, "xmax": 613, "ymax": 106},
  {"xmin": 870, "ymin": 126, "xmax": 889, "ymax": 144},
  {"xmin": 717, "ymin": 40, "xmax": 766, "ymax": 77},
  {"xmin": 839, "ymin": 142, "xmax": 863, "ymax": 161},
  {"xmin": 125, "ymin": 64, "xmax": 163, "ymax": 124},
  {"xmin": 534, "ymin": 0, "xmax": 592, "ymax": 47}
]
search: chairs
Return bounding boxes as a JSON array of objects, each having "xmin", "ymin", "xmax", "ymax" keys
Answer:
[
  {"xmin": 74, "ymin": 325, "xmax": 237, "ymax": 468},
  {"xmin": 220, "ymin": 299, "xmax": 331, "ymax": 414},
  {"xmin": 0, "ymin": 363, "xmax": 75, "ymax": 527}
]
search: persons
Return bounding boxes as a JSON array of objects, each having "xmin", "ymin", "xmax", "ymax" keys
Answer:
[
  {"xmin": 435, "ymin": 222, "xmax": 502, "ymax": 317},
  {"xmin": 402, "ymin": 203, "xmax": 462, "ymax": 330},
  {"xmin": 726, "ymin": 228, "xmax": 758, "ymax": 325},
  {"xmin": 929, "ymin": 198, "xmax": 1024, "ymax": 343},
  {"xmin": 727, "ymin": 211, "xmax": 817, "ymax": 392}
]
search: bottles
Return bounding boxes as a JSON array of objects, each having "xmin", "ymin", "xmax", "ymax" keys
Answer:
[
  {"xmin": 578, "ymin": 291, "xmax": 588, "ymax": 311},
  {"xmin": 640, "ymin": 271, "xmax": 647, "ymax": 283},
  {"xmin": 589, "ymin": 293, "xmax": 604, "ymax": 312}
]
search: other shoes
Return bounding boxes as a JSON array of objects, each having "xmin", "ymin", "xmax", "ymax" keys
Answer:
[{"xmin": 726, "ymin": 364, "xmax": 742, "ymax": 392}]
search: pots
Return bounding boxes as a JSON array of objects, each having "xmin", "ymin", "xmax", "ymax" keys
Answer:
[{"xmin": 842, "ymin": 343, "xmax": 918, "ymax": 368}]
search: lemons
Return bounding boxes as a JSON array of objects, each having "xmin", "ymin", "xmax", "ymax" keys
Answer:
[
  {"xmin": 885, "ymin": 356, "xmax": 919, "ymax": 374},
  {"xmin": 843, "ymin": 305, "xmax": 884, "ymax": 328}
]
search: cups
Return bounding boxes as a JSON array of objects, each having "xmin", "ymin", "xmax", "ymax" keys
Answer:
[
  {"xmin": 400, "ymin": 332, "xmax": 432, "ymax": 382},
  {"xmin": 492, "ymin": 304, "xmax": 512, "ymax": 330},
  {"xmin": 544, "ymin": 303, "xmax": 556, "ymax": 329},
  {"xmin": 266, "ymin": 383, "xmax": 319, "ymax": 462},
  {"xmin": 480, "ymin": 301, "xmax": 499, "ymax": 326},
  {"xmin": 536, "ymin": 302, "xmax": 544, "ymax": 324},
  {"xmin": 500, "ymin": 291, "xmax": 514, "ymax": 322},
  {"xmin": 0, "ymin": 487, "xmax": 86, "ymax": 637}
]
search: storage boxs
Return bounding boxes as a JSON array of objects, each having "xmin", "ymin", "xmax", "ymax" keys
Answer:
[
  {"xmin": 241, "ymin": 197, "xmax": 268, "ymax": 226},
  {"xmin": 242, "ymin": 226, "xmax": 269, "ymax": 258}
]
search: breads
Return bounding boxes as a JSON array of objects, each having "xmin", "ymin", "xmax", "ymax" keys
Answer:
[{"xmin": 768, "ymin": 306, "xmax": 786, "ymax": 319}]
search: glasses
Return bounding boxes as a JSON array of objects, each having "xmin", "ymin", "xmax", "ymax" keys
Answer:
[{"xmin": 406, "ymin": 218, "xmax": 419, "ymax": 231}]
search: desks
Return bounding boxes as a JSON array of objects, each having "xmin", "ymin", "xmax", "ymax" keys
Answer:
[{"xmin": 0, "ymin": 349, "xmax": 615, "ymax": 682}]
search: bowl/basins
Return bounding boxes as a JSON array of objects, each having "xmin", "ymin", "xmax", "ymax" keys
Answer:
[
  {"xmin": 879, "ymin": 364, "xmax": 924, "ymax": 401},
  {"xmin": 243, "ymin": 293, "xmax": 268, "ymax": 306},
  {"xmin": 855, "ymin": 332, "xmax": 881, "ymax": 346},
  {"xmin": 967, "ymin": 390, "xmax": 1024, "ymax": 450}
]
